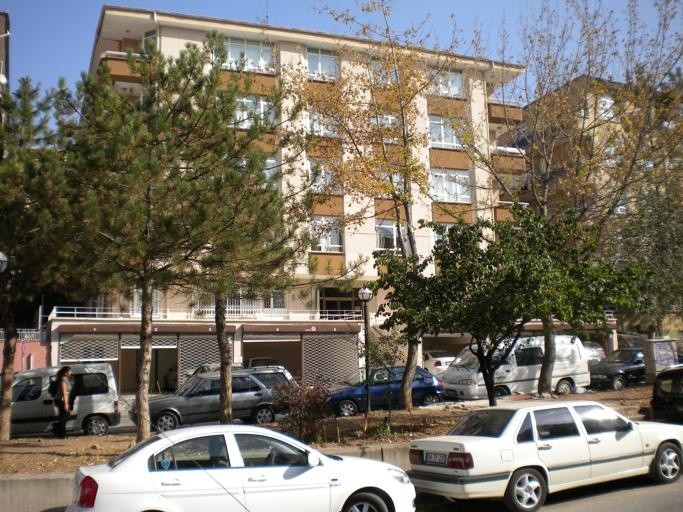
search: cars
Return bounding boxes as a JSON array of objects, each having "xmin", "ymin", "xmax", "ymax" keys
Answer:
[
  {"xmin": 422, "ymin": 349, "xmax": 456, "ymax": 375},
  {"xmin": 649, "ymin": 363, "xmax": 682, "ymax": 426},
  {"xmin": 588, "ymin": 348, "xmax": 646, "ymax": 391},
  {"xmin": 580, "ymin": 334, "xmax": 649, "ymax": 367},
  {"xmin": 304, "ymin": 364, "xmax": 443, "ymax": 420},
  {"xmin": 405, "ymin": 399, "xmax": 683, "ymax": 511},
  {"xmin": 244, "ymin": 357, "xmax": 286, "ymax": 368},
  {"xmin": 183, "ymin": 363, "xmax": 244, "ymax": 376},
  {"xmin": 128, "ymin": 373, "xmax": 275, "ymax": 433},
  {"xmin": 65, "ymin": 424, "xmax": 416, "ymax": 512}
]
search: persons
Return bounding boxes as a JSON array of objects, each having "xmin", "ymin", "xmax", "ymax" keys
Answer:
[{"xmin": 45, "ymin": 366, "xmax": 73, "ymax": 439}]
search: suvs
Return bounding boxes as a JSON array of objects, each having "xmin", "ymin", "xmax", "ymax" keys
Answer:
[{"xmin": 232, "ymin": 365, "xmax": 300, "ymax": 415}]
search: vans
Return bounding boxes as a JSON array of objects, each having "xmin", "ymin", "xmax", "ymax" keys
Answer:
[
  {"xmin": 442, "ymin": 335, "xmax": 591, "ymax": 400},
  {"xmin": 8, "ymin": 362, "xmax": 121, "ymax": 436}
]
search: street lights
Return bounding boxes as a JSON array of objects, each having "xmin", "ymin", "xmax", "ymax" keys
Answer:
[
  {"xmin": 357, "ymin": 285, "xmax": 373, "ymax": 416},
  {"xmin": 0, "ymin": 251, "xmax": 8, "ymax": 277}
]
